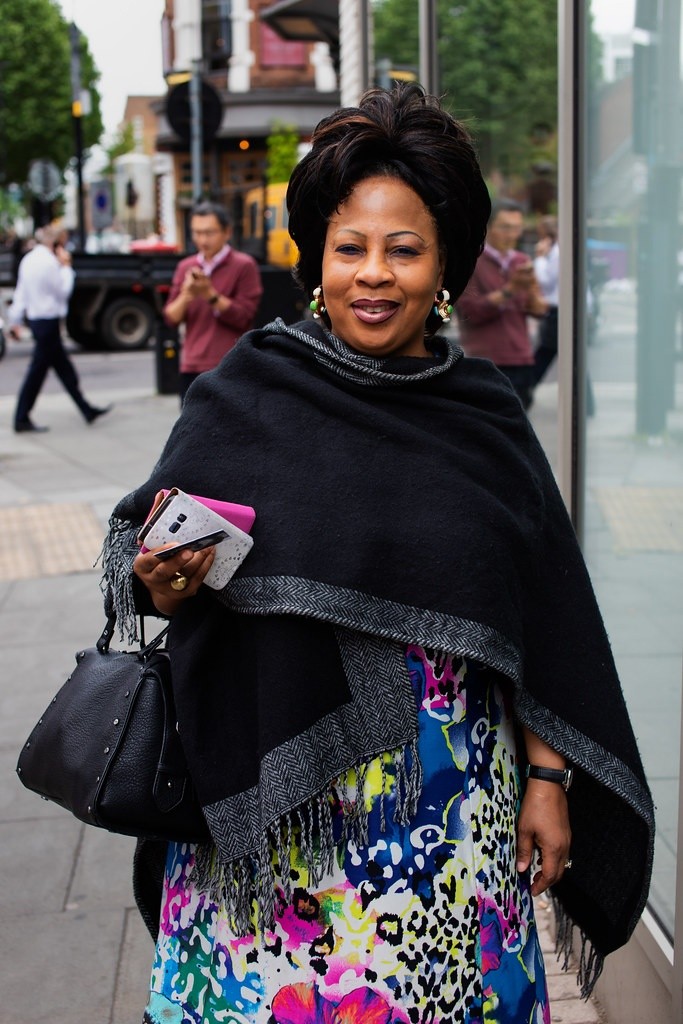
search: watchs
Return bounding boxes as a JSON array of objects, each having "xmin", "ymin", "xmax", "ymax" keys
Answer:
[{"xmin": 525, "ymin": 761, "xmax": 573, "ymax": 792}]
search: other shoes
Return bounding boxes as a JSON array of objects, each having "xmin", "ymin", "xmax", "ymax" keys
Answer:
[
  {"xmin": 15, "ymin": 423, "xmax": 50, "ymax": 433},
  {"xmin": 88, "ymin": 403, "xmax": 114, "ymax": 423}
]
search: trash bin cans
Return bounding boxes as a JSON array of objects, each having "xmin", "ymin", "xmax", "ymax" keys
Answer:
[{"xmin": 155, "ymin": 326, "xmax": 179, "ymax": 394}]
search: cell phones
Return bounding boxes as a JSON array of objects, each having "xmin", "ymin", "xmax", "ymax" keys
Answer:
[{"xmin": 138, "ymin": 487, "xmax": 253, "ymax": 589}]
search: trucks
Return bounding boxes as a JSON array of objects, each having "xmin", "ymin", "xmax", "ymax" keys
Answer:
[{"xmin": 0, "ymin": 177, "xmax": 298, "ymax": 350}]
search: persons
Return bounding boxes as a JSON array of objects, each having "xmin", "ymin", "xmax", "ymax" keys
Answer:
[
  {"xmin": 161, "ymin": 201, "xmax": 262, "ymax": 420},
  {"xmin": 8, "ymin": 213, "xmax": 113, "ymax": 433},
  {"xmin": 94, "ymin": 83, "xmax": 657, "ymax": 1024},
  {"xmin": 525, "ymin": 221, "xmax": 597, "ymax": 417},
  {"xmin": 457, "ymin": 195, "xmax": 546, "ymax": 408}
]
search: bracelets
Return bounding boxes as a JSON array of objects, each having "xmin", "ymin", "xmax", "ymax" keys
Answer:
[{"xmin": 206, "ymin": 293, "xmax": 222, "ymax": 306}]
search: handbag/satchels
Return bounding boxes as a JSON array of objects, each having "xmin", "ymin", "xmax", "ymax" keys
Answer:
[{"xmin": 16, "ymin": 599, "xmax": 210, "ymax": 844}]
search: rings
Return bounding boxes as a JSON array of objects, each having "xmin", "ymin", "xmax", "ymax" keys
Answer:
[
  {"xmin": 169, "ymin": 572, "xmax": 188, "ymax": 592},
  {"xmin": 564, "ymin": 860, "xmax": 573, "ymax": 869}
]
